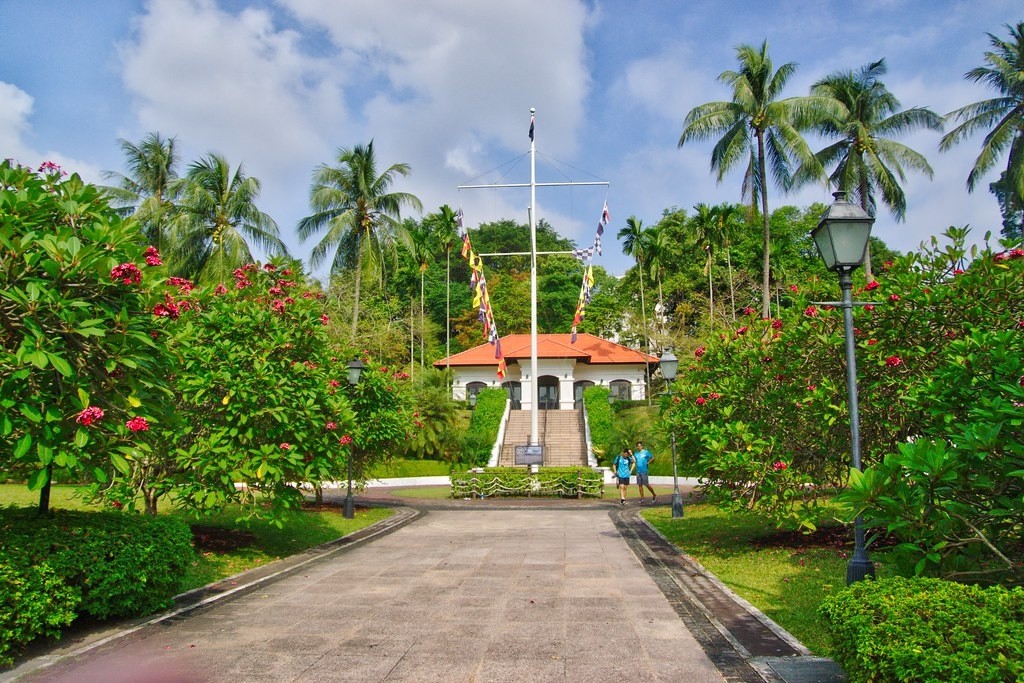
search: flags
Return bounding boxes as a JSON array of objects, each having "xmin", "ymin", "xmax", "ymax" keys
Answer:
[
  {"xmin": 571, "ymin": 203, "xmax": 610, "ymax": 345},
  {"xmin": 456, "ymin": 207, "xmax": 507, "ymax": 379}
]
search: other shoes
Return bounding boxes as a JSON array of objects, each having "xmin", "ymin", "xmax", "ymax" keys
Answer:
[
  {"xmin": 652, "ymin": 497, "xmax": 659, "ymax": 505},
  {"xmin": 637, "ymin": 499, "xmax": 645, "ymax": 504},
  {"xmin": 620, "ymin": 501, "xmax": 629, "ymax": 506}
]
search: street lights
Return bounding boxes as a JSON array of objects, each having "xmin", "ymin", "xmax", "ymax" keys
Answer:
[
  {"xmin": 342, "ymin": 358, "xmax": 367, "ymax": 520},
  {"xmin": 659, "ymin": 347, "xmax": 685, "ymax": 518},
  {"xmin": 811, "ymin": 191, "xmax": 876, "ymax": 590}
]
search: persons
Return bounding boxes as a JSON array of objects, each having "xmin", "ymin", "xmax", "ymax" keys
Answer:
[
  {"xmin": 613, "ymin": 448, "xmax": 635, "ymax": 506},
  {"xmin": 630, "ymin": 442, "xmax": 657, "ymax": 504}
]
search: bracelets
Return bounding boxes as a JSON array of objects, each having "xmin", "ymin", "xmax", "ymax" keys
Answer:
[{"xmin": 630, "ymin": 454, "xmax": 633, "ymax": 457}]
